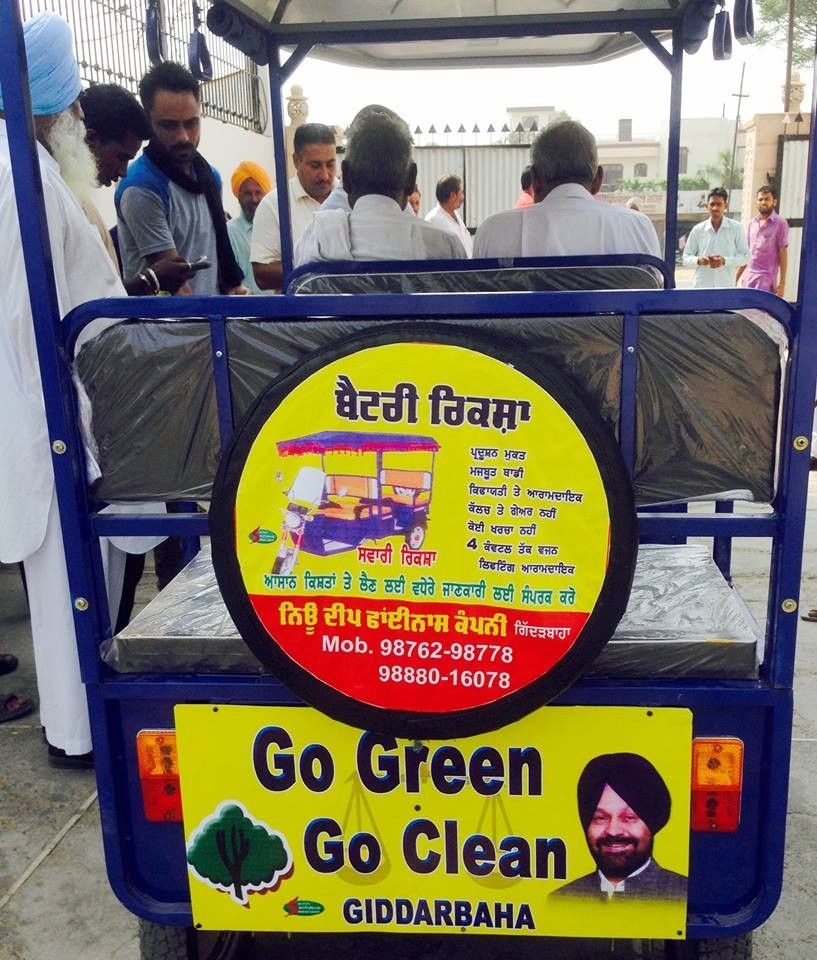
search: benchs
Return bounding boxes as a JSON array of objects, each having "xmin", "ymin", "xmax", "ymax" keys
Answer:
[
  {"xmin": 62, "ymin": 257, "xmax": 798, "ymax": 679},
  {"xmin": 315, "ymin": 473, "xmax": 380, "ymax": 522},
  {"xmin": 379, "ymin": 469, "xmax": 432, "ymax": 506}
]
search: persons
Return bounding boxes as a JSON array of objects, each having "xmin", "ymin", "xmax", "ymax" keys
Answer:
[
  {"xmin": 114, "ymin": 59, "xmax": 255, "ymax": 591},
  {"xmin": 512, "ymin": 164, "xmax": 534, "ymax": 209},
  {"xmin": 546, "ymin": 752, "xmax": 687, "ymax": 908},
  {"xmin": 295, "ymin": 104, "xmax": 467, "ymax": 269},
  {"xmin": 0, "ymin": 654, "xmax": 34, "ymax": 723},
  {"xmin": 682, "ymin": 187, "xmax": 750, "ymax": 288},
  {"xmin": 225, "ymin": 160, "xmax": 280, "ymax": 296},
  {"xmin": 471, "ymin": 121, "xmax": 662, "ymax": 259},
  {"xmin": 627, "ymin": 196, "xmax": 643, "ymax": 212},
  {"xmin": 249, "ymin": 123, "xmax": 341, "ymax": 296},
  {"xmin": 738, "ymin": 186, "xmax": 789, "ymax": 297},
  {"xmin": 0, "ymin": 13, "xmax": 168, "ymax": 770},
  {"xmin": 322, "ymin": 170, "xmax": 474, "ymax": 259},
  {"xmin": 79, "ymin": 83, "xmax": 212, "ymax": 638}
]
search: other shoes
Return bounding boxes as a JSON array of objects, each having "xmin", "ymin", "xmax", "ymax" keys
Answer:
[
  {"xmin": 47, "ymin": 745, "xmax": 93, "ymax": 770},
  {"xmin": 42, "ymin": 726, "xmax": 53, "ymax": 746},
  {"xmin": 0, "ymin": 693, "xmax": 33, "ymax": 722},
  {"xmin": 0, "ymin": 652, "xmax": 17, "ymax": 674}
]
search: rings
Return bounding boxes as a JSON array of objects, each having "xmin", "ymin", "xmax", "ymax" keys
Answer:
[{"xmin": 186, "ymin": 263, "xmax": 192, "ymax": 271}]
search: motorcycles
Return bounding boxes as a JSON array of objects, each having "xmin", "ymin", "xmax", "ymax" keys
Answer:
[{"xmin": 0, "ymin": 1, "xmax": 816, "ymax": 960}]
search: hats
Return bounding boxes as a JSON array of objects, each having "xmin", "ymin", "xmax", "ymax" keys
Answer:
[
  {"xmin": 231, "ymin": 161, "xmax": 271, "ymax": 197},
  {"xmin": 578, "ymin": 753, "xmax": 670, "ymax": 833},
  {"xmin": 0, "ymin": 10, "xmax": 83, "ymax": 115}
]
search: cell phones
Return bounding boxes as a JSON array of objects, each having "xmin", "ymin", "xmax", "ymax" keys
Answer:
[{"xmin": 189, "ymin": 255, "xmax": 207, "ymax": 265}]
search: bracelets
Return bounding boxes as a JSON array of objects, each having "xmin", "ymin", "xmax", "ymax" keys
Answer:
[
  {"xmin": 145, "ymin": 267, "xmax": 160, "ymax": 295},
  {"xmin": 136, "ymin": 272, "xmax": 156, "ymax": 296}
]
